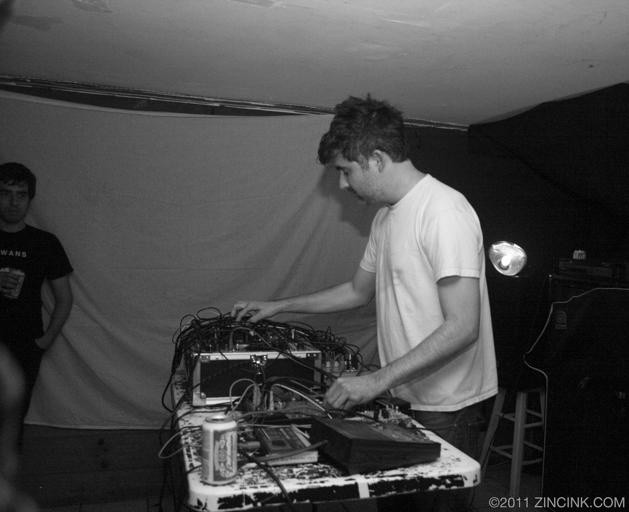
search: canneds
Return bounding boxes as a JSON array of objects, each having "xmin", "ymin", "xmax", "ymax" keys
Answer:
[
  {"xmin": 3, "ymin": 268, "xmax": 26, "ymax": 299},
  {"xmin": 201, "ymin": 413, "xmax": 239, "ymax": 484}
]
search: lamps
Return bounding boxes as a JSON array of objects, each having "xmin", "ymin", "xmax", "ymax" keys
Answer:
[{"xmin": 487, "ymin": 238, "xmax": 529, "ymax": 278}]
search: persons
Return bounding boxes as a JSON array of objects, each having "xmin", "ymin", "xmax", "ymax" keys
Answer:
[
  {"xmin": 0, "ymin": 161, "xmax": 74, "ymax": 470},
  {"xmin": 232, "ymin": 95, "xmax": 501, "ymax": 512}
]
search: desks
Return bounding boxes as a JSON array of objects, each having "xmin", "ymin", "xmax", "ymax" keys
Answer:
[{"xmin": 169, "ymin": 366, "xmax": 483, "ymax": 512}]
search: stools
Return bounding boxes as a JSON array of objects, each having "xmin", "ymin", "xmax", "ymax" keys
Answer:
[{"xmin": 478, "ymin": 366, "xmax": 551, "ymax": 502}]
